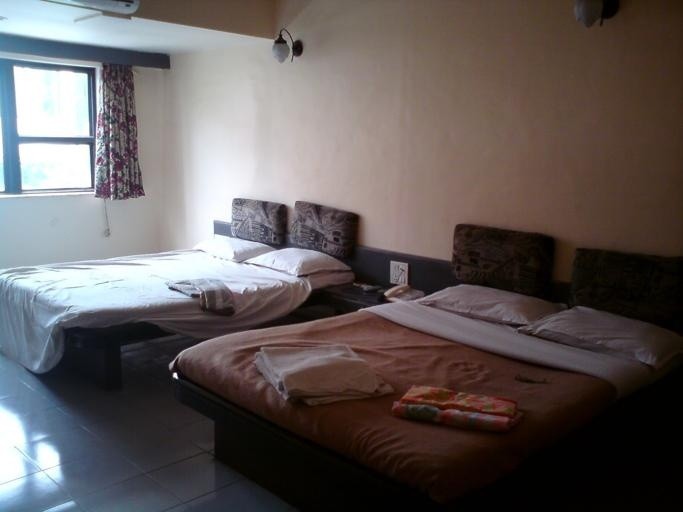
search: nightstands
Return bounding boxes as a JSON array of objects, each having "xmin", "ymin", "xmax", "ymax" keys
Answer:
[{"xmin": 322, "ymin": 284, "xmax": 388, "ymax": 315}]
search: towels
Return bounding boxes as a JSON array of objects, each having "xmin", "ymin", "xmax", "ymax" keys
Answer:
[{"xmin": 252, "ymin": 342, "xmax": 394, "ymax": 407}]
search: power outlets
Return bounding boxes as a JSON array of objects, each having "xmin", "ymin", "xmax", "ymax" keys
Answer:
[{"xmin": 389, "ymin": 261, "xmax": 399, "ymax": 284}]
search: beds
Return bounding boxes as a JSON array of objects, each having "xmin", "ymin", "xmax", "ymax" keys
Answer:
[
  {"xmin": 159, "ymin": 218, "xmax": 682, "ymax": 512},
  {"xmin": 0, "ymin": 196, "xmax": 360, "ymax": 390}
]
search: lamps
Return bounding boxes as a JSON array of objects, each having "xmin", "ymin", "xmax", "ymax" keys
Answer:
[
  {"xmin": 271, "ymin": 27, "xmax": 303, "ymax": 64},
  {"xmin": 570, "ymin": 0, "xmax": 622, "ymax": 28}
]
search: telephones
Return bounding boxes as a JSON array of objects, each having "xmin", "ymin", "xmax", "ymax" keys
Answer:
[{"xmin": 383, "ymin": 284, "xmax": 425, "ymax": 303}]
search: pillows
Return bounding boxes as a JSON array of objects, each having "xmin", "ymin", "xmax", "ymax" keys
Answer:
[
  {"xmin": 519, "ymin": 302, "xmax": 682, "ymax": 371},
  {"xmin": 191, "ymin": 232, "xmax": 276, "ymax": 265},
  {"xmin": 243, "ymin": 246, "xmax": 352, "ymax": 276},
  {"xmin": 418, "ymin": 283, "xmax": 569, "ymax": 327}
]
